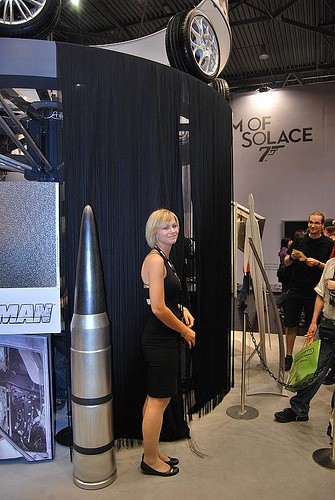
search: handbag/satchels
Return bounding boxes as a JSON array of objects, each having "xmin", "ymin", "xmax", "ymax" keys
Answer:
[
  {"xmin": 277, "ymin": 265, "xmax": 286, "ymax": 282},
  {"xmin": 284, "ymin": 336, "xmax": 321, "ymax": 392},
  {"xmin": 318, "ymin": 320, "xmax": 335, "ymax": 344}
]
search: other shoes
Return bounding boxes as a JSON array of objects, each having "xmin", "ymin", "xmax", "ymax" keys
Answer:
[
  {"xmin": 237, "ymin": 303, "xmax": 247, "ymax": 309},
  {"xmin": 142, "ymin": 453, "xmax": 179, "ymax": 466},
  {"xmin": 141, "ymin": 460, "xmax": 179, "ymax": 477},
  {"xmin": 285, "ymin": 355, "xmax": 293, "ymax": 371}
]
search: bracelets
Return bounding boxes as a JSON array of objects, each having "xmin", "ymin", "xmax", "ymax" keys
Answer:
[{"xmin": 316, "ymin": 263, "xmax": 320, "ymax": 267}]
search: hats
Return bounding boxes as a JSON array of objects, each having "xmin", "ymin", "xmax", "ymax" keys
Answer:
[{"xmin": 324, "ymin": 218, "xmax": 335, "ymax": 229}]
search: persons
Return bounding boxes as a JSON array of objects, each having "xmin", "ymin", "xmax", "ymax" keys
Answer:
[
  {"xmin": 141, "ymin": 210, "xmax": 196, "ymax": 477},
  {"xmin": 237, "ymin": 263, "xmax": 255, "ymax": 309},
  {"xmin": 277, "ymin": 238, "xmax": 292, "ymax": 307},
  {"xmin": 275, "ymin": 257, "xmax": 335, "ymax": 436},
  {"xmin": 278, "ymin": 211, "xmax": 334, "ymax": 371},
  {"xmin": 295, "ymin": 230, "xmax": 304, "ymax": 238},
  {"xmin": 323, "ymin": 218, "xmax": 335, "ymax": 258}
]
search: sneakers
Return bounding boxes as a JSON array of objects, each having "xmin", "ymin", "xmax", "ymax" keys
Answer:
[{"xmin": 274, "ymin": 407, "xmax": 309, "ymax": 423}]
